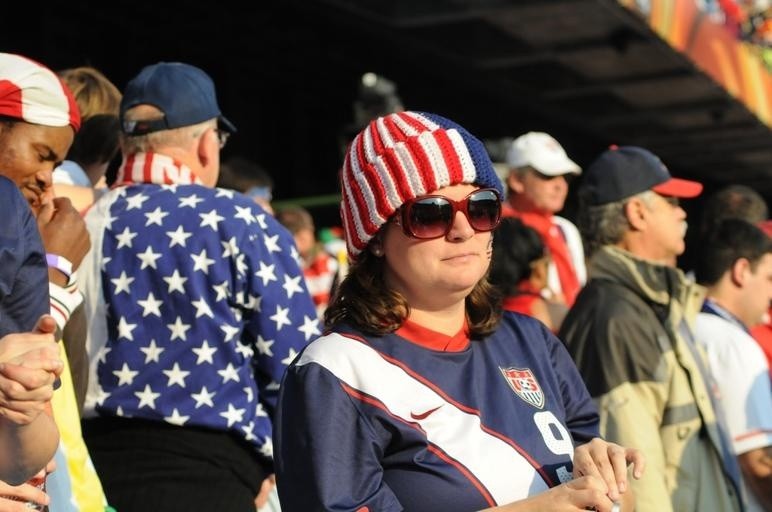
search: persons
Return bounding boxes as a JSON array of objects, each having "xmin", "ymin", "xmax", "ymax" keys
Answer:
[
  {"xmin": 278, "ymin": 111, "xmax": 771, "ymax": 511},
  {"xmin": 1, "ymin": 61, "xmax": 316, "ymax": 511}
]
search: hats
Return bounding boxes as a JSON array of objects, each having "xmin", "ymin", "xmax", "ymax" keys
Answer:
[
  {"xmin": 338, "ymin": 109, "xmax": 506, "ymax": 265},
  {"xmin": 577, "ymin": 141, "xmax": 705, "ymax": 206},
  {"xmin": 119, "ymin": 61, "xmax": 239, "ymax": 138},
  {"xmin": 507, "ymin": 130, "xmax": 585, "ymax": 179}
]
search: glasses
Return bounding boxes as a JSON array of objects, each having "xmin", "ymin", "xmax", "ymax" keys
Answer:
[{"xmin": 390, "ymin": 186, "xmax": 503, "ymax": 240}]
